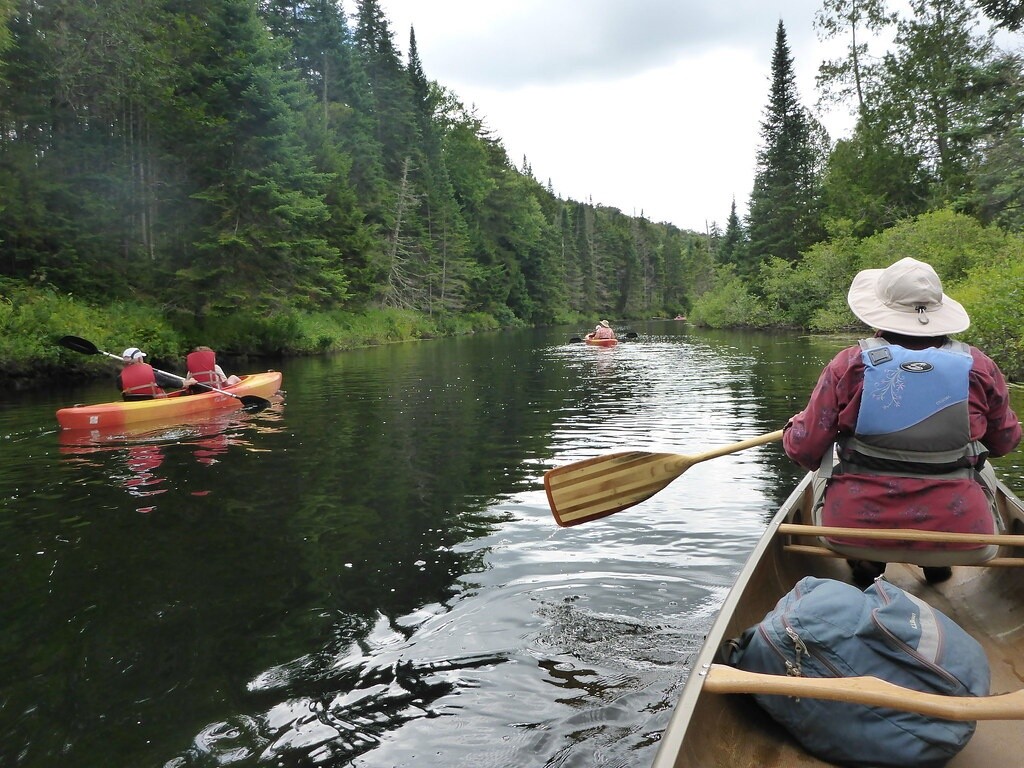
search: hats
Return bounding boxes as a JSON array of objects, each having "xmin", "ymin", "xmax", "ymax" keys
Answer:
[
  {"xmin": 847, "ymin": 258, "xmax": 971, "ymax": 338},
  {"xmin": 599, "ymin": 320, "xmax": 609, "ymax": 328},
  {"xmin": 123, "ymin": 347, "xmax": 147, "ymax": 361}
]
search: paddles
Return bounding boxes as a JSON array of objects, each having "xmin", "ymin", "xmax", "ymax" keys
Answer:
[
  {"xmin": 545, "ymin": 428, "xmax": 785, "ymax": 526},
  {"xmin": 570, "ymin": 332, "xmax": 638, "ymax": 344},
  {"xmin": 59, "ymin": 333, "xmax": 269, "ymax": 407}
]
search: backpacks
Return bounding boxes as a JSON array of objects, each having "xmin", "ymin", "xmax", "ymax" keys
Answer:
[{"xmin": 722, "ymin": 575, "xmax": 991, "ymax": 767}]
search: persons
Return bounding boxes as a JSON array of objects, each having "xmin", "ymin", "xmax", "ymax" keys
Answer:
[
  {"xmin": 115, "ymin": 347, "xmax": 198, "ymax": 399},
  {"xmin": 186, "ymin": 346, "xmax": 243, "ymax": 385},
  {"xmin": 588, "ymin": 319, "xmax": 616, "ymax": 339},
  {"xmin": 780, "ymin": 256, "xmax": 1021, "ymax": 590}
]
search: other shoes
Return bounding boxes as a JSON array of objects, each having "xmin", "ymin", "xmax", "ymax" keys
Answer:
[
  {"xmin": 923, "ymin": 565, "xmax": 951, "ymax": 582},
  {"xmin": 847, "ymin": 555, "xmax": 880, "ymax": 583}
]
